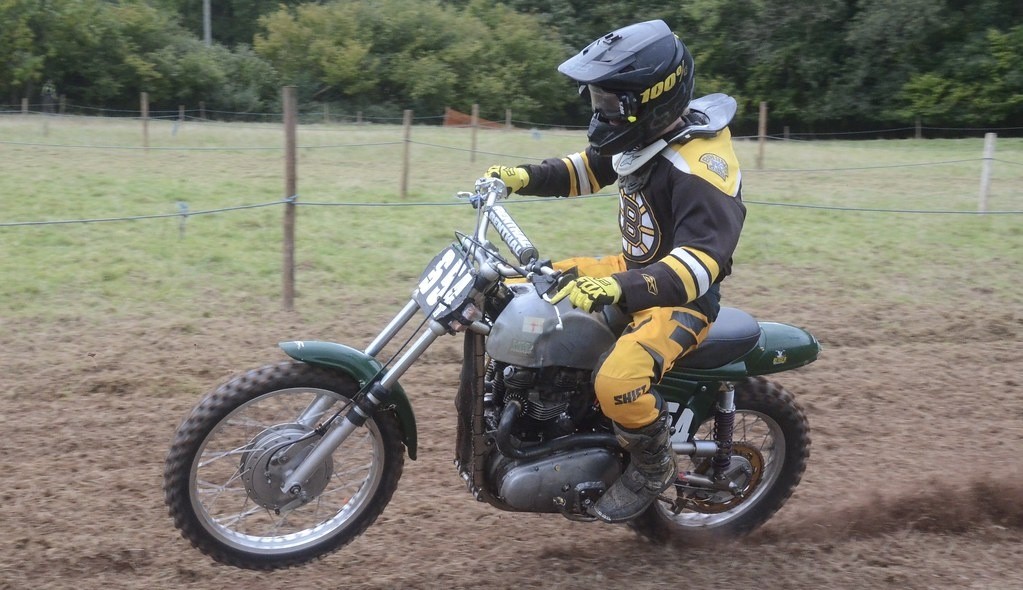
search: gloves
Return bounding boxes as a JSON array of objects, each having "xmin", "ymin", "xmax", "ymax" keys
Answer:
[
  {"xmin": 483, "ymin": 164, "xmax": 533, "ymax": 199},
  {"xmin": 550, "ymin": 276, "xmax": 625, "ymax": 314}
]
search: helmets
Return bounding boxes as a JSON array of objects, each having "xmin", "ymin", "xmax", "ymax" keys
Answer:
[{"xmin": 557, "ymin": 20, "xmax": 695, "ymax": 156}]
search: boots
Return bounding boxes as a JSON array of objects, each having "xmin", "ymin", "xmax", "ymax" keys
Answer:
[{"xmin": 590, "ymin": 392, "xmax": 678, "ymax": 524}]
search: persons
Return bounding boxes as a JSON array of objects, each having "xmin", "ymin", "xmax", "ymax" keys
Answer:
[{"xmin": 476, "ymin": 19, "xmax": 747, "ymax": 525}]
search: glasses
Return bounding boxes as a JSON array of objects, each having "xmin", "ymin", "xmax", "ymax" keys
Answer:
[{"xmin": 588, "ymin": 84, "xmax": 622, "ymax": 119}]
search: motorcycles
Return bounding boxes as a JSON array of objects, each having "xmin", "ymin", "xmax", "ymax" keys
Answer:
[{"xmin": 162, "ymin": 177, "xmax": 819, "ymax": 573}]
